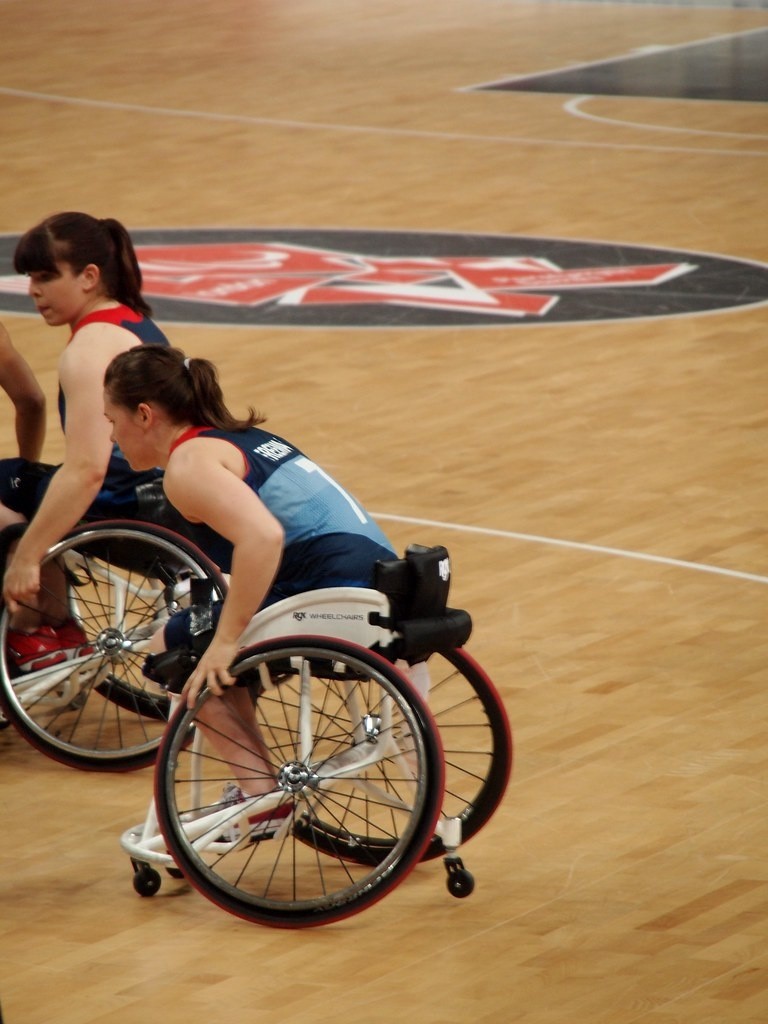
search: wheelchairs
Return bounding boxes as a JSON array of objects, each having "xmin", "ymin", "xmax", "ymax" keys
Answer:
[
  {"xmin": 1, "ymin": 468, "xmax": 241, "ymax": 776},
  {"xmin": 118, "ymin": 540, "xmax": 515, "ymax": 935}
]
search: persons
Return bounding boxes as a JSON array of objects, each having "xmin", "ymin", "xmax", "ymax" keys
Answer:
[
  {"xmin": 0, "ymin": 324, "xmax": 47, "ymax": 463},
  {"xmin": 0, "ymin": 211, "xmax": 172, "ymax": 676},
  {"xmin": 101, "ymin": 343, "xmax": 399, "ymax": 843}
]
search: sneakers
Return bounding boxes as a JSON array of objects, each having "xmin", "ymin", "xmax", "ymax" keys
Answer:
[
  {"xmin": 210, "ymin": 781, "xmax": 295, "ymax": 841},
  {"xmin": 4, "ymin": 621, "xmax": 66, "ymax": 671},
  {"xmin": 51, "ymin": 620, "xmax": 93, "ymax": 661}
]
